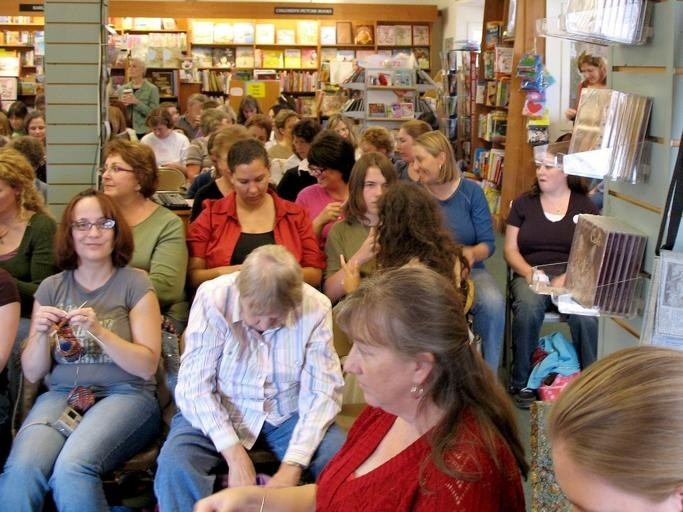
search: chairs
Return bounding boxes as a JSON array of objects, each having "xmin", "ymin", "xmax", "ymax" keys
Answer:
[
  {"xmin": 503, "ymin": 254, "xmax": 568, "ymax": 373},
  {"xmin": 529, "ymin": 402, "xmax": 579, "ymax": 511},
  {"xmin": 18, "ymin": 331, "xmax": 173, "ymax": 510},
  {"xmin": 154, "ymin": 165, "xmax": 183, "ymax": 194},
  {"xmin": 329, "ymin": 309, "xmax": 354, "ymax": 357}
]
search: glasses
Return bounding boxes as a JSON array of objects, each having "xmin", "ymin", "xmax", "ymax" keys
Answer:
[
  {"xmin": 308, "ymin": 164, "xmax": 328, "ymax": 175},
  {"xmin": 96, "ymin": 165, "xmax": 135, "ymax": 175},
  {"xmin": 72, "ymin": 219, "xmax": 115, "ymax": 231}
]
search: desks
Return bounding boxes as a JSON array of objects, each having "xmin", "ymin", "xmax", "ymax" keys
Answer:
[{"xmin": 160, "ymin": 197, "xmax": 195, "ymax": 243}]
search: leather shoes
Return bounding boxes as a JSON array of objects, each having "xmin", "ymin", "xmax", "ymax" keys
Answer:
[{"xmin": 510, "ymin": 382, "xmax": 537, "ymax": 409}]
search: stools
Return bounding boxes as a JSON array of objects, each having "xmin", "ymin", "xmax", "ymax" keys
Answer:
[{"xmin": 222, "ymin": 441, "xmax": 279, "ymax": 474}]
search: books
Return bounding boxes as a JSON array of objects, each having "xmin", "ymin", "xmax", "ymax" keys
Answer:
[
  {"xmin": 0, "ymin": 14, "xmax": 43, "ymax": 25},
  {"xmin": 192, "ymin": 19, "xmax": 429, "ymax": 45},
  {"xmin": 108, "ymin": 13, "xmax": 176, "ymax": 30}
]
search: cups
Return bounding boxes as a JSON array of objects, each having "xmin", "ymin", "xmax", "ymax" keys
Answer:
[{"xmin": 124, "ymin": 88, "xmax": 134, "ymax": 96}]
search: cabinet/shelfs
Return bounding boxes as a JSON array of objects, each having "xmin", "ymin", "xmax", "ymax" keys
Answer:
[
  {"xmin": 440, "ymin": 35, "xmax": 546, "ymax": 232},
  {"xmin": 0, "ymin": 17, "xmax": 45, "ymax": 113},
  {"xmin": 106, "ymin": 20, "xmax": 441, "ymax": 128}
]
